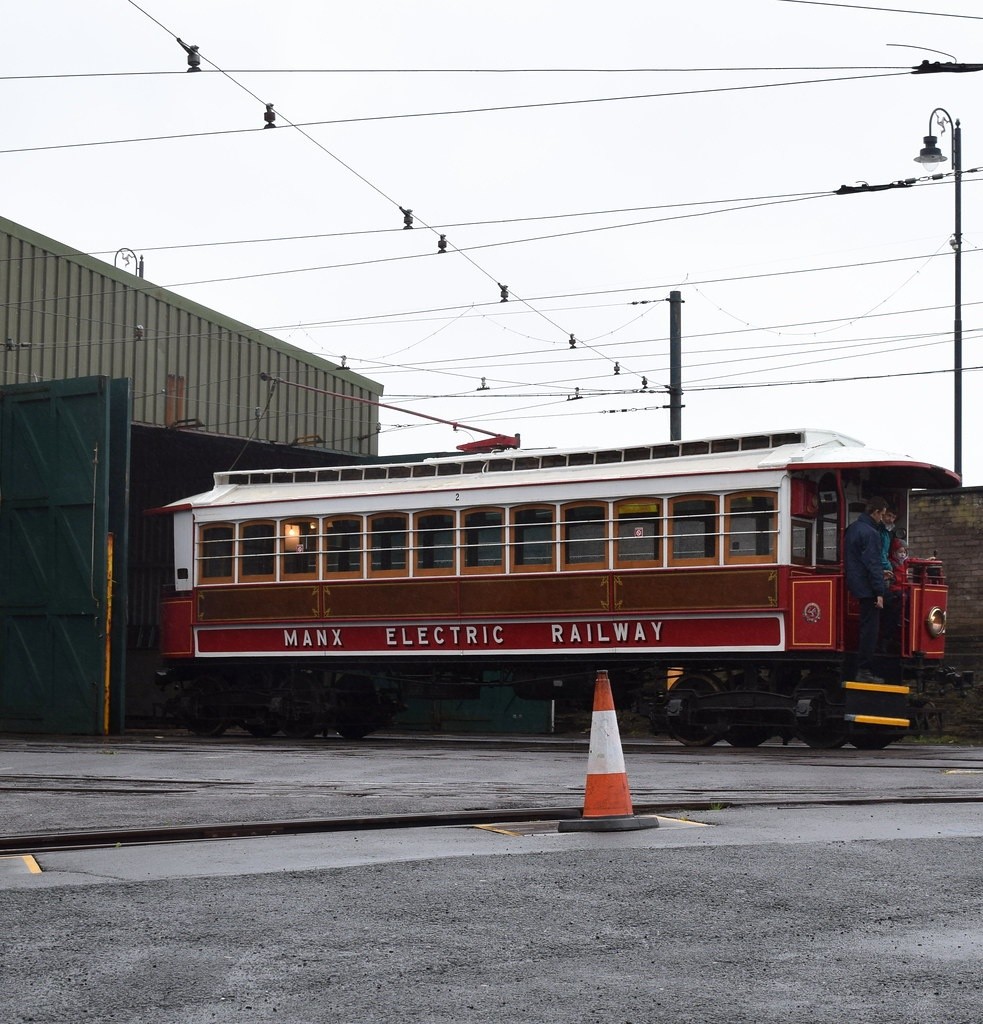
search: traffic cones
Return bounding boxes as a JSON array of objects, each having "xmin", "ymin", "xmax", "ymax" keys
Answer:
[{"xmin": 559, "ymin": 666, "xmax": 665, "ymax": 840}]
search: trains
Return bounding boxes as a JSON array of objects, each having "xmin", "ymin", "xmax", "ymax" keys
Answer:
[{"xmin": 142, "ymin": 428, "xmax": 969, "ymax": 753}]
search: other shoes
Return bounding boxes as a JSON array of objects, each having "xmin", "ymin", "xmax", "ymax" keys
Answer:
[{"xmin": 857, "ymin": 668, "xmax": 885, "ymax": 685}]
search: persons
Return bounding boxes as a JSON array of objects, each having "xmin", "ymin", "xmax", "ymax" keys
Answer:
[
  {"xmin": 876, "ymin": 502, "xmax": 909, "ymax": 653},
  {"xmin": 888, "ymin": 538, "xmax": 910, "ymax": 653},
  {"xmin": 844, "ymin": 496, "xmax": 889, "ymax": 684}
]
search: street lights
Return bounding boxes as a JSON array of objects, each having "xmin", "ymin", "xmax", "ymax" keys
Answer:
[{"xmin": 912, "ymin": 108, "xmax": 967, "ymax": 488}]
